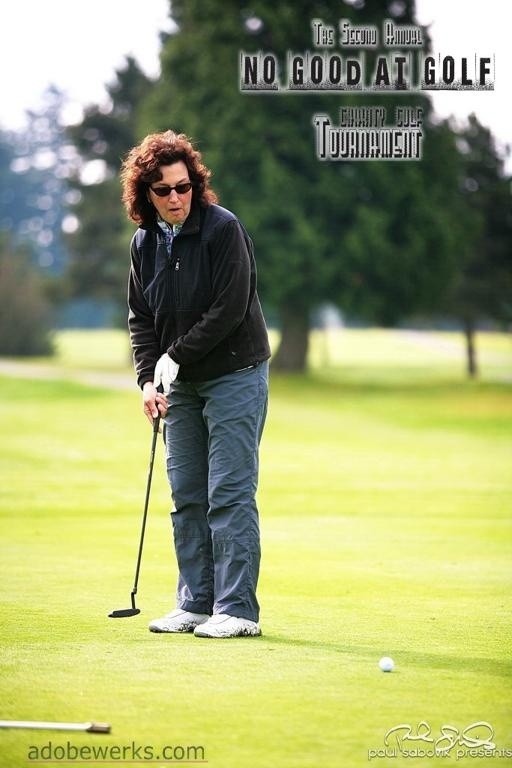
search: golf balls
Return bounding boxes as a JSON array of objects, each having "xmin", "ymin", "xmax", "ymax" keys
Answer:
[{"xmin": 378, "ymin": 657, "xmax": 396, "ymax": 673}]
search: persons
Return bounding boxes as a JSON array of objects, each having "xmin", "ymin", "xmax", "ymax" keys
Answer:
[{"xmin": 121, "ymin": 129, "xmax": 271, "ymax": 637}]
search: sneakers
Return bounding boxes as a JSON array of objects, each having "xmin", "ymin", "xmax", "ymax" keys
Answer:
[
  {"xmin": 148, "ymin": 609, "xmax": 209, "ymax": 633},
  {"xmin": 194, "ymin": 613, "xmax": 262, "ymax": 638}
]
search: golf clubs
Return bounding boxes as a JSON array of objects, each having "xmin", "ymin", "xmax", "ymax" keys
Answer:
[{"xmin": 107, "ymin": 384, "xmax": 164, "ymax": 617}]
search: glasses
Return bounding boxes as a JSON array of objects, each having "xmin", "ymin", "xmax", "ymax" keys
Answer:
[{"xmin": 148, "ymin": 183, "xmax": 192, "ymax": 197}]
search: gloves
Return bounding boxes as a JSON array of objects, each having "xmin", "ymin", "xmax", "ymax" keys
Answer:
[{"xmin": 152, "ymin": 353, "xmax": 180, "ymax": 393}]
